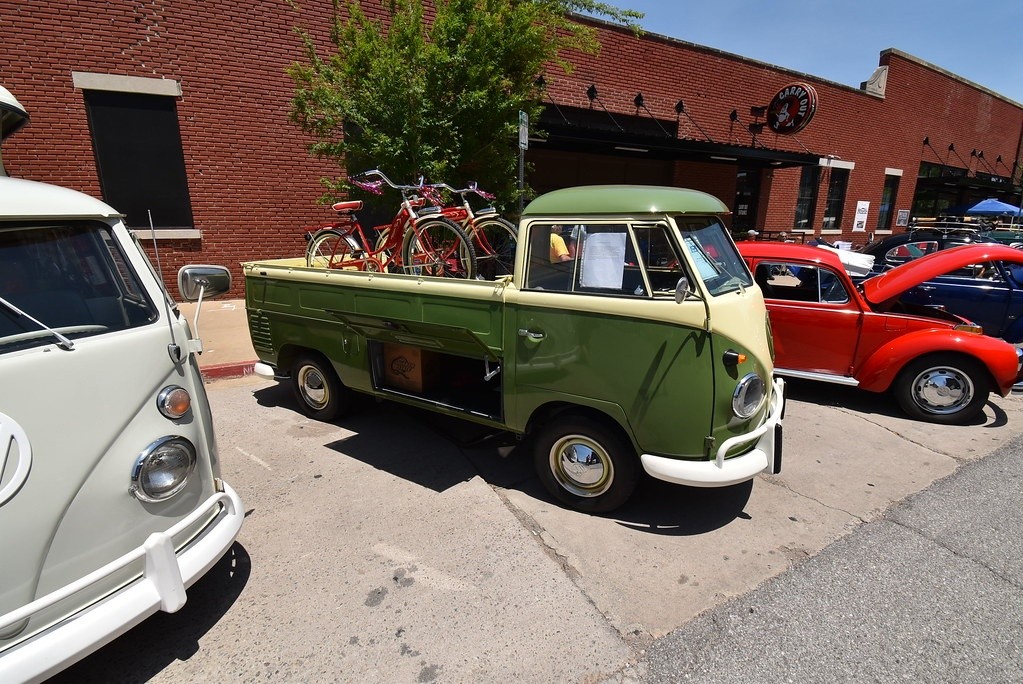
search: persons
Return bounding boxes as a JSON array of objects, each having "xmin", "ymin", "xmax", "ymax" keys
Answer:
[{"xmin": 545, "ymin": 225, "xmax": 576, "ymax": 265}]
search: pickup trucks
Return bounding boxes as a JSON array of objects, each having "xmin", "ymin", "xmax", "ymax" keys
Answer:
[
  {"xmin": 237, "ymin": 182, "xmax": 786, "ymax": 516},
  {"xmin": 0, "ymin": 82, "xmax": 260, "ymax": 684}
]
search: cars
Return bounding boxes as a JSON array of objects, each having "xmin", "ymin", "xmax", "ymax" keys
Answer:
[
  {"xmin": 942, "ymin": 226, "xmax": 1023, "ymax": 252},
  {"xmin": 665, "ymin": 239, "xmax": 1023, "ymax": 423},
  {"xmin": 830, "ymin": 219, "xmax": 1023, "ymax": 348}
]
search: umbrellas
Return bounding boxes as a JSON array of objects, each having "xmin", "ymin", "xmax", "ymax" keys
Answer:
[{"xmin": 965, "ymin": 196, "xmax": 1022, "ymax": 223}]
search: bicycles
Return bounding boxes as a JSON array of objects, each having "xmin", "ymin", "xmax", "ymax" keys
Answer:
[
  {"xmin": 302, "ymin": 170, "xmax": 476, "ymax": 285},
  {"xmin": 373, "ymin": 181, "xmax": 521, "ymax": 286}
]
